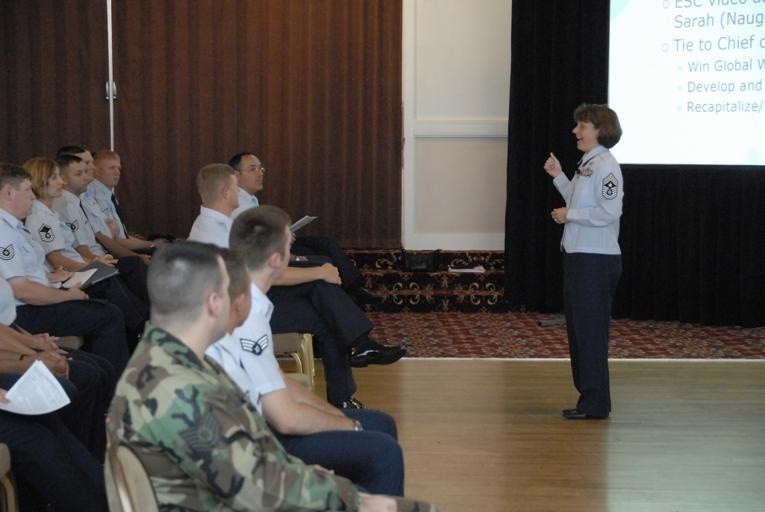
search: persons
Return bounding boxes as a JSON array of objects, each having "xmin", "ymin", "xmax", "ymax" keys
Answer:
[
  {"xmin": 544, "ymin": 103, "xmax": 624, "ymax": 421},
  {"xmin": 102, "ymin": 236, "xmax": 438, "ymax": 512},
  {"xmin": 0, "ymin": 143, "xmax": 187, "ymax": 510},
  {"xmin": 228, "ymin": 153, "xmax": 386, "ymax": 311},
  {"xmin": 187, "ymin": 164, "xmax": 405, "ymax": 412},
  {"xmin": 203, "ymin": 247, "xmax": 268, "ymax": 419},
  {"xmin": 218, "ymin": 205, "xmax": 405, "ymax": 494}
]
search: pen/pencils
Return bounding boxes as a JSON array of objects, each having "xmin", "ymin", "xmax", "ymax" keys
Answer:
[{"xmin": 64, "ymin": 357, "xmax": 74, "ymax": 361}]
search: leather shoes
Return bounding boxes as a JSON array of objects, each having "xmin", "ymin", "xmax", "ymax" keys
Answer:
[
  {"xmin": 562, "ymin": 409, "xmax": 587, "ymax": 420},
  {"xmin": 352, "ymin": 286, "xmax": 383, "ymax": 304},
  {"xmin": 333, "ymin": 398, "xmax": 367, "ymax": 410},
  {"xmin": 349, "ymin": 340, "xmax": 407, "ymax": 368}
]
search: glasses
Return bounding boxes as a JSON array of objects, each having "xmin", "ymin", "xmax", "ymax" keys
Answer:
[{"xmin": 239, "ymin": 167, "xmax": 266, "ymax": 173}]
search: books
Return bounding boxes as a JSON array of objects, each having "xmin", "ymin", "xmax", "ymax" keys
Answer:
[{"xmin": 447, "ymin": 265, "xmax": 486, "ymax": 274}]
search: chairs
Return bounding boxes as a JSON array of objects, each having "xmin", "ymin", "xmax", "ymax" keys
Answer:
[{"xmin": 103, "ymin": 434, "xmax": 161, "ymax": 512}]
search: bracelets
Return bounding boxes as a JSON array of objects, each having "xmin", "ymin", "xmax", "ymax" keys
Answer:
[
  {"xmin": 293, "ymin": 254, "xmax": 301, "ymax": 262},
  {"xmin": 353, "ymin": 417, "xmax": 361, "ymax": 431}
]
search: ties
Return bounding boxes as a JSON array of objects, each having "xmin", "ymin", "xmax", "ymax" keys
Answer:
[{"xmin": 111, "ymin": 194, "xmax": 126, "ymax": 224}]
